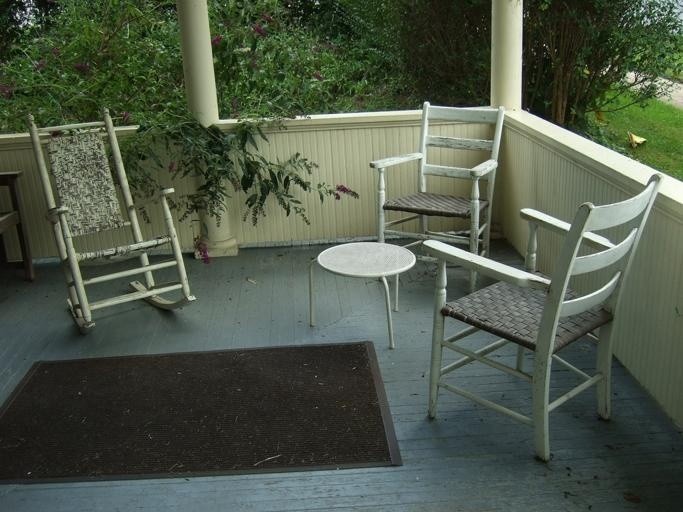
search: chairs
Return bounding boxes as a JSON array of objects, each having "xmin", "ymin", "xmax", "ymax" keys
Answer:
[
  {"xmin": 421, "ymin": 173, "xmax": 665, "ymax": 464},
  {"xmin": 27, "ymin": 105, "xmax": 199, "ymax": 337},
  {"xmin": 369, "ymin": 98, "xmax": 505, "ymax": 295}
]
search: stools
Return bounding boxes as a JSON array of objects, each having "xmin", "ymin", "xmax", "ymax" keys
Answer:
[{"xmin": 307, "ymin": 241, "xmax": 415, "ymax": 350}]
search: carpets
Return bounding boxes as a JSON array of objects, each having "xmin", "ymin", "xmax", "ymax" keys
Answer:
[{"xmin": 1, "ymin": 340, "xmax": 403, "ymax": 486}]
